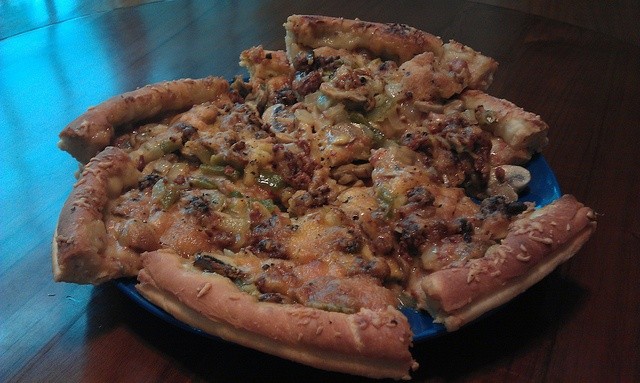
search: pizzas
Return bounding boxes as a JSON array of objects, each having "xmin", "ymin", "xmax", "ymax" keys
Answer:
[{"xmin": 51, "ymin": 14, "xmax": 601, "ymax": 380}]
